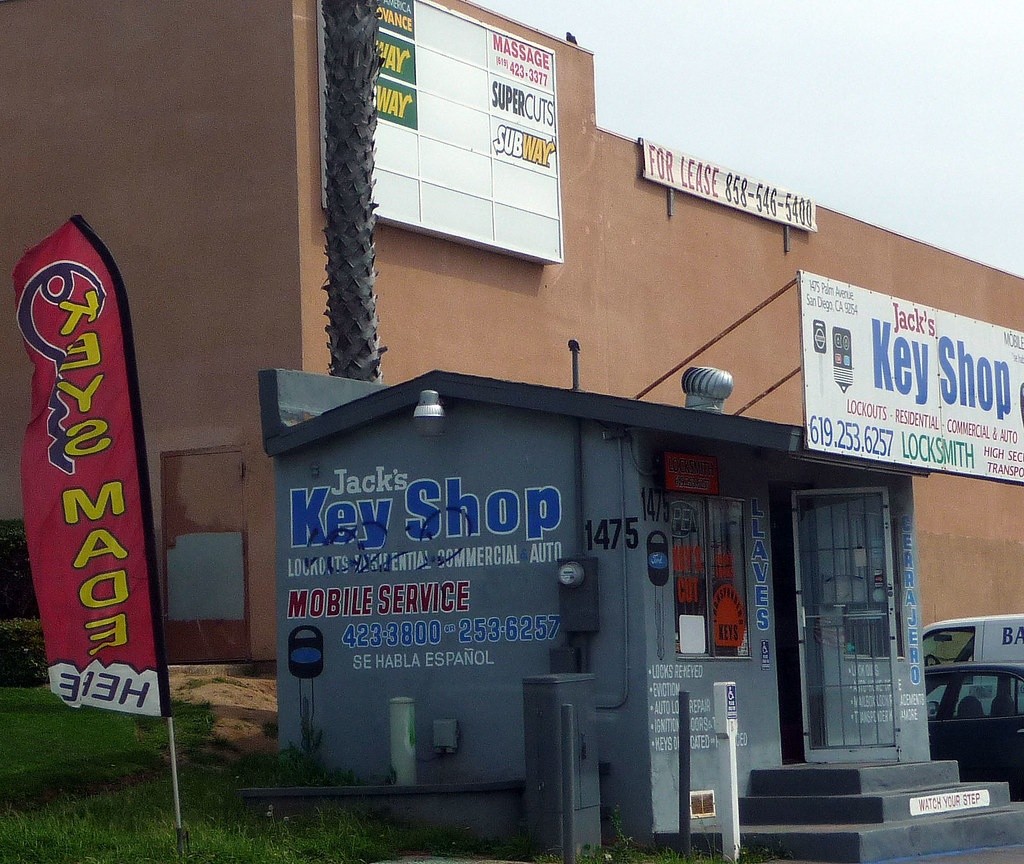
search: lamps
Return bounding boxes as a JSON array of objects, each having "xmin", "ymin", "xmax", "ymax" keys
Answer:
[
  {"xmin": 431, "ymin": 717, "xmax": 460, "ymax": 753},
  {"xmin": 413, "ymin": 389, "xmax": 446, "ymax": 439}
]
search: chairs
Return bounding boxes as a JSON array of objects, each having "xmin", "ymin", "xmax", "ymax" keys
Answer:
[
  {"xmin": 989, "ymin": 693, "xmax": 1016, "ymax": 717},
  {"xmin": 956, "ymin": 696, "xmax": 983, "ymax": 719}
]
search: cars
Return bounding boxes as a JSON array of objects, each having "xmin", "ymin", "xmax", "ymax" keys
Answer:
[{"xmin": 923, "ymin": 662, "xmax": 1024, "ymax": 804}]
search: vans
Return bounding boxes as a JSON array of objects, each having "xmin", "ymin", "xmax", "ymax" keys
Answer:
[{"xmin": 920, "ymin": 613, "xmax": 1024, "ymax": 722}]
